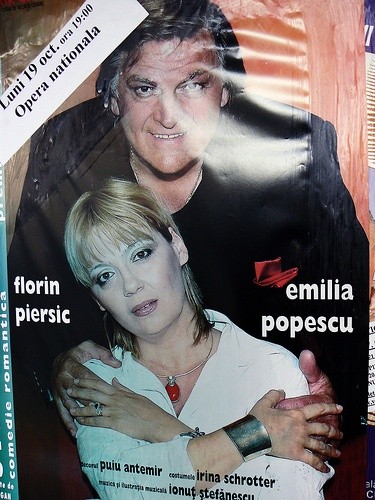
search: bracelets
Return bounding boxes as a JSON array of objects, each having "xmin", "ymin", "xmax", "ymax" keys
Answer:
[{"xmin": 222, "ymin": 413, "xmax": 273, "ymax": 465}]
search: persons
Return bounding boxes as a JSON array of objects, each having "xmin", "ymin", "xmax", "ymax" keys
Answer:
[
  {"xmin": 7, "ymin": 0, "xmax": 373, "ymax": 498},
  {"xmin": 63, "ymin": 176, "xmax": 343, "ymax": 499}
]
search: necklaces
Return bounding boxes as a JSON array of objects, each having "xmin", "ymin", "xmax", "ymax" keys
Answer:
[
  {"xmin": 154, "ymin": 328, "xmax": 214, "ymax": 404},
  {"xmin": 126, "ymin": 148, "xmax": 204, "ymax": 212}
]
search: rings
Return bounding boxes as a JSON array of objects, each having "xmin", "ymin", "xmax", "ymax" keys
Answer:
[{"xmin": 94, "ymin": 402, "xmax": 102, "ymax": 416}]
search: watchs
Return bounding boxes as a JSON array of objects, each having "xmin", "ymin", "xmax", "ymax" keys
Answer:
[{"xmin": 30, "ymin": 348, "xmax": 65, "ymax": 398}]
